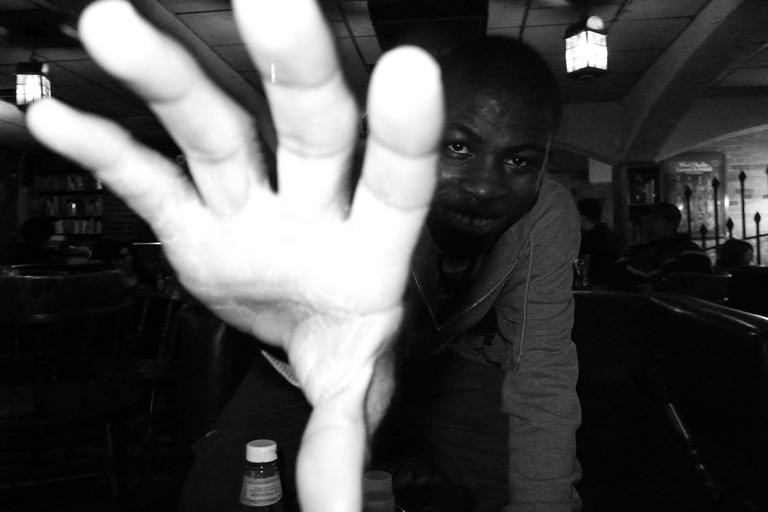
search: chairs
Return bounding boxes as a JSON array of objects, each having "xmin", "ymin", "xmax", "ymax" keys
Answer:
[
  {"xmin": 566, "ymin": 261, "xmax": 768, "ymax": 501},
  {"xmin": 2, "ymin": 257, "xmax": 187, "ymax": 407}
]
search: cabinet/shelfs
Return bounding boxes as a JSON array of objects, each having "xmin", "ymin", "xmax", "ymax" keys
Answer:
[{"xmin": 25, "ymin": 170, "xmax": 109, "ymax": 237}]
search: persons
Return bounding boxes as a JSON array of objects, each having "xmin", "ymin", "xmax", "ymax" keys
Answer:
[
  {"xmin": 715, "ymin": 238, "xmax": 752, "ymax": 266},
  {"xmin": 609, "ymin": 200, "xmax": 712, "ymax": 292},
  {"xmin": 25, "ymin": 0, "xmax": 591, "ymax": 511},
  {"xmin": 110, "ymin": 241, "xmax": 155, "ymax": 288},
  {"xmin": 576, "ymin": 195, "xmax": 618, "ymax": 288}
]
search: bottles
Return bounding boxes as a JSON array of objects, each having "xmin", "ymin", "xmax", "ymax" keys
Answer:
[{"xmin": 236, "ymin": 439, "xmax": 286, "ymax": 512}]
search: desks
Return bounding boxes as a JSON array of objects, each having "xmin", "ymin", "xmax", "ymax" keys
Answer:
[{"xmin": 0, "ymin": 355, "xmax": 142, "ymax": 512}]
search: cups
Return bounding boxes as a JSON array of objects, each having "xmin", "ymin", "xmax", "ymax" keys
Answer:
[{"xmin": 575, "ymin": 255, "xmax": 592, "ymax": 287}]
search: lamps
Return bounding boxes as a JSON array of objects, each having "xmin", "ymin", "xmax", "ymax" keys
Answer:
[
  {"xmin": 11, "ymin": 32, "xmax": 57, "ymax": 105},
  {"xmin": 564, "ymin": 14, "xmax": 608, "ymax": 83}
]
